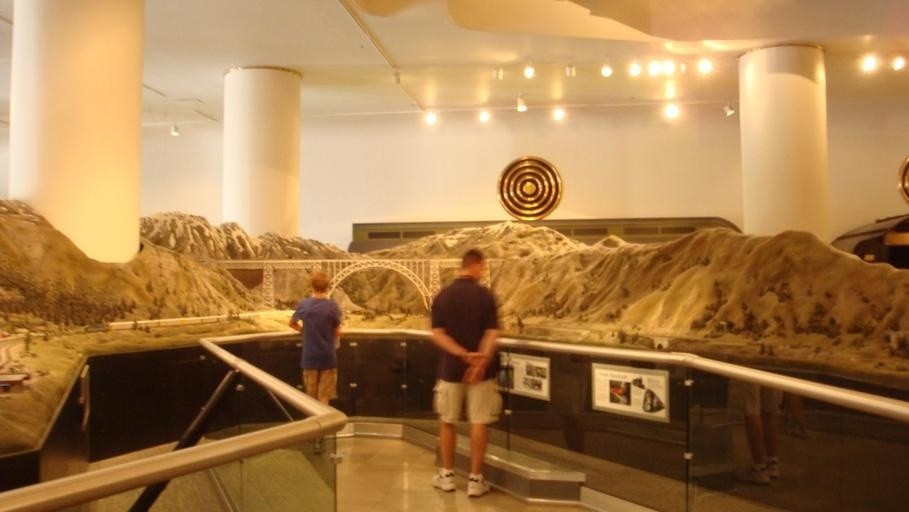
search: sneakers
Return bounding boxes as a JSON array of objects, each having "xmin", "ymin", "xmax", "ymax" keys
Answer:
[
  {"xmin": 432, "ymin": 468, "xmax": 457, "ymax": 491},
  {"xmin": 313, "ymin": 436, "xmax": 326, "ymax": 453},
  {"xmin": 467, "ymin": 472, "xmax": 491, "ymax": 497}
]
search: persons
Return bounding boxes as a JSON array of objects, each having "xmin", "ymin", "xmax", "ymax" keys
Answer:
[
  {"xmin": 432, "ymin": 248, "xmax": 502, "ymax": 497},
  {"xmin": 725, "ymin": 369, "xmax": 807, "ymax": 486},
  {"xmin": 289, "ymin": 274, "xmax": 342, "ymax": 454}
]
